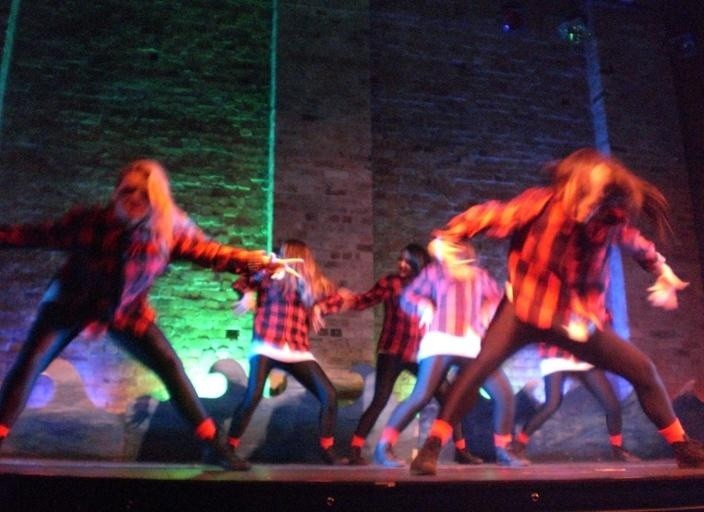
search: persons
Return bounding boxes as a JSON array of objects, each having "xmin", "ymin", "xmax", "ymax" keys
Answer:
[
  {"xmin": 372, "ymin": 236, "xmax": 515, "ymax": 471},
  {"xmin": 0, "ymin": 159, "xmax": 304, "ymax": 473},
  {"xmin": 513, "ymin": 339, "xmax": 644, "ymax": 465},
  {"xmin": 344, "ymin": 242, "xmax": 487, "ymax": 466},
  {"xmin": 408, "ymin": 148, "xmax": 704, "ymax": 476},
  {"xmin": 220, "ymin": 239, "xmax": 349, "ymax": 472}
]
